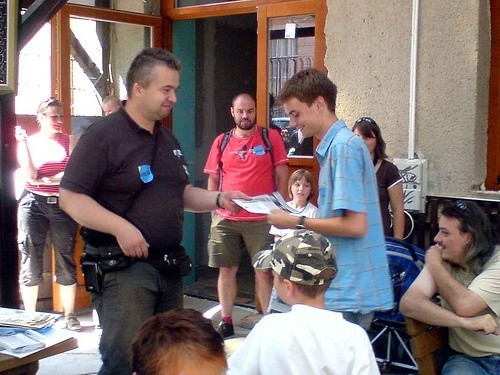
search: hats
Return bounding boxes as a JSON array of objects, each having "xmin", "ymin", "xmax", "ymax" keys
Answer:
[{"xmin": 252, "ymin": 229, "xmax": 338, "ymax": 286}]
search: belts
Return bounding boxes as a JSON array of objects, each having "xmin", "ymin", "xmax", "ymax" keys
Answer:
[{"xmin": 24, "ymin": 190, "xmax": 59, "ymax": 205}]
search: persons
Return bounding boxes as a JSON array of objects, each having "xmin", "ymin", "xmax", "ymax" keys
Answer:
[
  {"xmin": 130, "ymin": 310, "xmax": 228, "ymax": 375},
  {"xmin": 269, "ymin": 169, "xmax": 317, "ymax": 242},
  {"xmin": 266, "ymin": 69, "xmax": 394, "ymax": 332},
  {"xmin": 203, "ymin": 93, "xmax": 289, "ymax": 338},
  {"xmin": 102, "ymin": 96, "xmax": 122, "ymax": 116},
  {"xmin": 399, "ymin": 201, "xmax": 500, "ymax": 375},
  {"xmin": 59, "ymin": 48, "xmax": 253, "ymax": 375},
  {"xmin": 352, "ymin": 117, "xmax": 404, "ymax": 239},
  {"xmin": 225, "ymin": 229, "xmax": 381, "ymax": 375},
  {"xmin": 14, "ymin": 97, "xmax": 83, "ymax": 330}
]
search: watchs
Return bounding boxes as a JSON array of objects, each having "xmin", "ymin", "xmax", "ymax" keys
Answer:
[{"xmin": 296, "ymin": 216, "xmax": 307, "ymax": 229}]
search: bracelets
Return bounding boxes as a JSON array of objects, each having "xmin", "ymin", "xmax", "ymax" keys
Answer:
[{"xmin": 216, "ymin": 192, "xmax": 224, "ymax": 209}]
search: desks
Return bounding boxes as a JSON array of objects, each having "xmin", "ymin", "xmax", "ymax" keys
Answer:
[{"xmin": 0, "ymin": 322, "xmax": 79, "ymax": 375}]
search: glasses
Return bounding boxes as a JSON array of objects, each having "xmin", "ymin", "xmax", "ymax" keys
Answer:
[
  {"xmin": 457, "ymin": 200, "xmax": 474, "ymax": 215},
  {"xmin": 357, "ymin": 118, "xmax": 376, "ymax": 124}
]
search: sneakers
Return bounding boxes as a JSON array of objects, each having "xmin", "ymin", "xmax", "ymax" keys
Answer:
[{"xmin": 215, "ymin": 320, "xmax": 234, "ymax": 337}]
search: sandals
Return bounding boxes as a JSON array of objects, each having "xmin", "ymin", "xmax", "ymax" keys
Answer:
[{"xmin": 65, "ymin": 314, "xmax": 83, "ymax": 332}]
